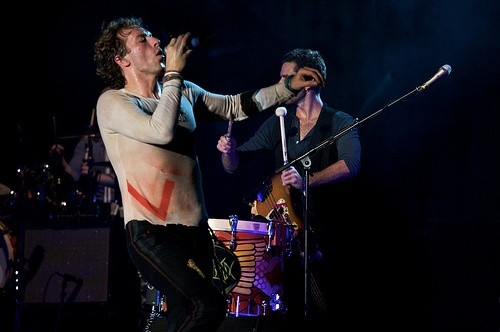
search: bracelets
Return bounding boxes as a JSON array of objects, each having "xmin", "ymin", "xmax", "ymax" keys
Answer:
[
  {"xmin": 162, "ymin": 74, "xmax": 183, "ymax": 84},
  {"xmin": 284, "ymin": 75, "xmax": 303, "ymax": 94}
]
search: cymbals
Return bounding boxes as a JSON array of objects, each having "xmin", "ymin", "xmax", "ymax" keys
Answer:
[
  {"xmin": 93, "ymin": 160, "xmax": 114, "ymax": 169},
  {"xmin": 54, "ymin": 126, "xmax": 101, "ymax": 140}
]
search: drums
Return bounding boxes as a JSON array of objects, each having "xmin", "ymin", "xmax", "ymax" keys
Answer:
[{"xmin": 206, "ymin": 216, "xmax": 295, "ymax": 318}]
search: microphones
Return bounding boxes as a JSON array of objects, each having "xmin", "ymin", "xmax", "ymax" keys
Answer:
[
  {"xmin": 162, "ymin": 36, "xmax": 204, "ymax": 57},
  {"xmin": 419, "ymin": 65, "xmax": 452, "ymax": 92}
]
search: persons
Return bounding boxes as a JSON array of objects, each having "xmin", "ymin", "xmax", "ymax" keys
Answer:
[
  {"xmin": 49, "ymin": 133, "xmax": 115, "ymax": 204},
  {"xmin": 216, "ymin": 48, "xmax": 362, "ymax": 265},
  {"xmin": 91, "ymin": 18, "xmax": 325, "ymax": 332}
]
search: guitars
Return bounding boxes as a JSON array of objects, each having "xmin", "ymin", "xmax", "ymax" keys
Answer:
[{"xmin": 248, "ymin": 174, "xmax": 333, "ymax": 318}]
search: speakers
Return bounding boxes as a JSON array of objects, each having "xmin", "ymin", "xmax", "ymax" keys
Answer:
[{"xmin": 8, "ymin": 216, "xmax": 133, "ymax": 311}]
